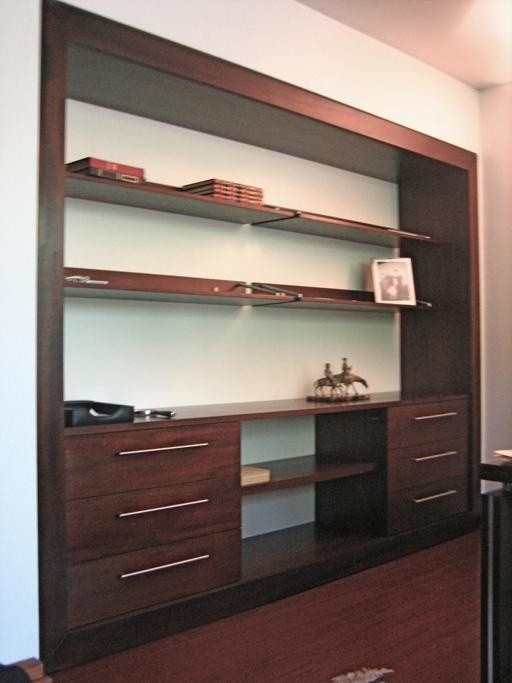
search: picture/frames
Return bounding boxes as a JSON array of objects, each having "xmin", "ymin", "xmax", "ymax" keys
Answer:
[{"xmin": 368, "ymin": 258, "xmax": 416, "ymax": 306}]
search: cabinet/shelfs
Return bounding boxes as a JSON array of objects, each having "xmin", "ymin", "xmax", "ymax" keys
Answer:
[{"xmin": 41, "ymin": 0, "xmax": 478, "ymax": 672}]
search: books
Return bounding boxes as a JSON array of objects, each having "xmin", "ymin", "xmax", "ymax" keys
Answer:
[
  {"xmin": 65, "ymin": 157, "xmax": 146, "ymax": 187},
  {"xmin": 182, "ymin": 178, "xmax": 264, "ymax": 206}
]
